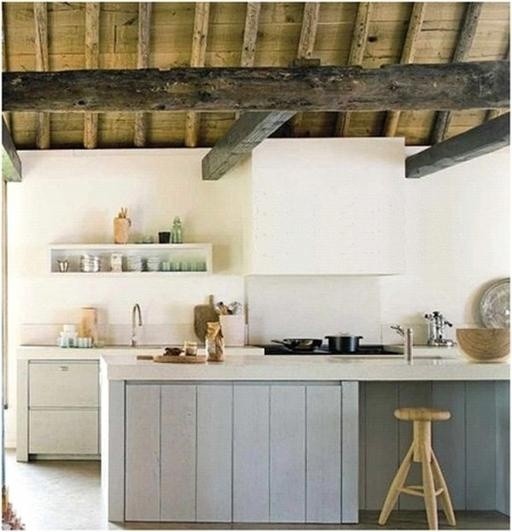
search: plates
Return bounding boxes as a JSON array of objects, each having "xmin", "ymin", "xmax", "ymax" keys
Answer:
[{"xmin": 479, "ymin": 277, "xmax": 510, "ymax": 329}]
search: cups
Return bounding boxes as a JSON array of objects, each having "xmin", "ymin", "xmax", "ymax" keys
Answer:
[
  {"xmin": 56, "ymin": 261, "xmax": 68, "ymax": 272},
  {"xmin": 158, "ymin": 231, "xmax": 171, "ymax": 243},
  {"xmin": 113, "ymin": 217, "xmax": 131, "ymax": 243},
  {"xmin": 109, "ymin": 253, "xmax": 160, "ymax": 272},
  {"xmin": 80, "ymin": 254, "xmax": 101, "ymax": 272},
  {"xmin": 161, "ymin": 261, "xmax": 206, "ymax": 271},
  {"xmin": 58, "ymin": 324, "xmax": 92, "ymax": 348},
  {"xmin": 79, "ymin": 307, "xmax": 97, "ymax": 346}
]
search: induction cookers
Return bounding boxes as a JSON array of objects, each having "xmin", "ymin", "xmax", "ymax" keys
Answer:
[{"xmin": 250, "ymin": 345, "xmax": 407, "ymax": 356}]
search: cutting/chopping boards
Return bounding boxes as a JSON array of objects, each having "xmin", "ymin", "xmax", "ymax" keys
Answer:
[
  {"xmin": 193, "ymin": 295, "xmax": 220, "ymax": 344},
  {"xmin": 136, "ymin": 349, "xmax": 208, "ymax": 364}
]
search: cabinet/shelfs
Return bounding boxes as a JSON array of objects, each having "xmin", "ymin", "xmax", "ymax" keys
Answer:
[
  {"xmin": 27, "ymin": 359, "xmax": 101, "ymax": 461},
  {"xmin": 48, "ymin": 242, "xmax": 214, "ymax": 276}
]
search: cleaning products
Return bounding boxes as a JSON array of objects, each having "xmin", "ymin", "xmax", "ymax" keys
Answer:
[{"xmin": 110, "ymin": 253, "xmax": 124, "ymax": 271}]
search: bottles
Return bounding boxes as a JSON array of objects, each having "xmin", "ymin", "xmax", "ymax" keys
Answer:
[
  {"xmin": 204, "ymin": 322, "xmax": 225, "ymax": 362},
  {"xmin": 171, "ymin": 216, "xmax": 183, "ymax": 244},
  {"xmin": 219, "ymin": 301, "xmax": 234, "ymax": 315}
]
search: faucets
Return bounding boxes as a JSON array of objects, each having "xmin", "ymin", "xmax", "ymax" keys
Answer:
[
  {"xmin": 131, "ymin": 303, "xmax": 143, "ymax": 337},
  {"xmin": 390, "ymin": 324, "xmax": 404, "ymax": 336}
]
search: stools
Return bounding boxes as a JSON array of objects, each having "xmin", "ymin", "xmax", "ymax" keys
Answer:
[{"xmin": 376, "ymin": 404, "xmax": 458, "ymax": 530}]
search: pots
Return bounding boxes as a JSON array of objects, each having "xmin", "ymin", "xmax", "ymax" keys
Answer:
[
  {"xmin": 324, "ymin": 330, "xmax": 363, "ymax": 352},
  {"xmin": 270, "ymin": 338, "xmax": 323, "ymax": 351}
]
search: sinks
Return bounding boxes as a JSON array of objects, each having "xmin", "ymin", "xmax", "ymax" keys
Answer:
[{"xmin": 337, "ymin": 354, "xmax": 457, "ymax": 361}]
